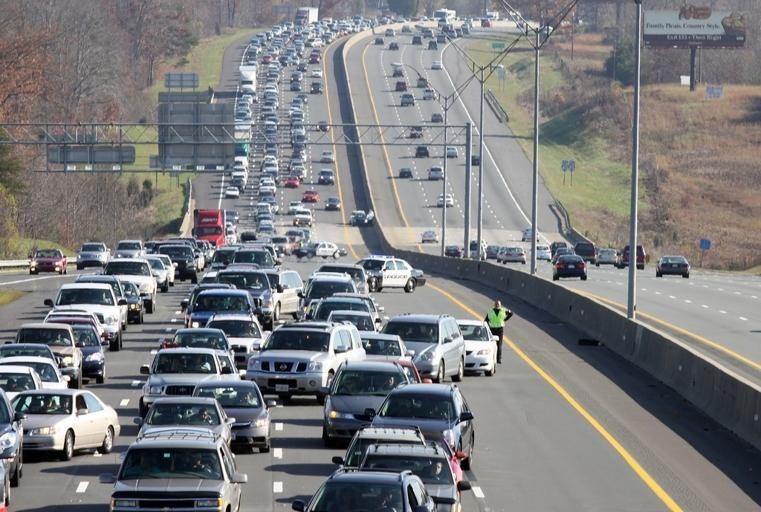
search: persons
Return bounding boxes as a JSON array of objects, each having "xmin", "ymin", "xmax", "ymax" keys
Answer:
[
  {"xmin": 60, "ymin": 397, "xmax": 72, "ymax": 415},
  {"xmin": 357, "ymin": 316, "xmax": 369, "ymax": 329},
  {"xmin": 202, "ymin": 297, "xmax": 211, "ymax": 308},
  {"xmin": 54, "ymin": 333, "xmax": 70, "ymax": 344},
  {"xmin": 241, "ymin": 390, "xmax": 255, "ymax": 407},
  {"xmin": 484, "ymin": 299, "xmax": 514, "ymax": 364},
  {"xmin": 78, "ymin": 333, "xmax": 89, "ymax": 343},
  {"xmin": 208, "ymin": 337, "xmax": 220, "ymax": 346},
  {"xmin": 424, "ymin": 326, "xmax": 435, "ymax": 341},
  {"xmin": 197, "ymin": 407, "xmax": 212, "ymax": 426},
  {"xmin": 471, "ymin": 327, "xmax": 484, "ymax": 341},
  {"xmin": 385, "ymin": 376, "xmax": 395, "ymax": 393},
  {"xmin": 426, "ymin": 460, "xmax": 444, "ymax": 480},
  {"xmin": 186, "ymin": 452, "xmax": 211, "ymax": 475},
  {"xmin": 40, "ymin": 396, "xmax": 54, "ymax": 412},
  {"xmin": 330, "ymin": 487, "xmax": 357, "ymax": 510},
  {"xmin": 235, "ymin": 299, "xmax": 244, "ymax": 311},
  {"xmin": 373, "ymin": 486, "xmax": 397, "ymax": 510}
]
